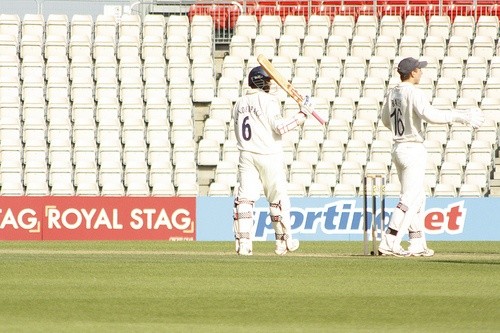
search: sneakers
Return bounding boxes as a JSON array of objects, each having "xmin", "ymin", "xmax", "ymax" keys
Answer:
[
  {"xmin": 274, "ymin": 239, "xmax": 300, "ymax": 256},
  {"xmin": 236, "ymin": 238, "xmax": 253, "ymax": 256},
  {"xmin": 377, "ymin": 231, "xmax": 412, "ymax": 258},
  {"xmin": 408, "ymin": 237, "xmax": 434, "ymax": 257}
]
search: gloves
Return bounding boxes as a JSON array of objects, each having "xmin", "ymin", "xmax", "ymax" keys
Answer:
[
  {"xmin": 299, "ymin": 95, "xmax": 316, "ymax": 118},
  {"xmin": 462, "ymin": 108, "xmax": 485, "ymax": 128}
]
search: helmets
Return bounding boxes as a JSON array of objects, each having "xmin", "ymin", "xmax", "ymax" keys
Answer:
[{"xmin": 248, "ymin": 66, "xmax": 272, "ymax": 93}]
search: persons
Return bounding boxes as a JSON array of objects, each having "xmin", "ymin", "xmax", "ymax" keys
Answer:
[
  {"xmin": 231, "ymin": 66, "xmax": 316, "ymax": 257},
  {"xmin": 379, "ymin": 56, "xmax": 482, "ymax": 257}
]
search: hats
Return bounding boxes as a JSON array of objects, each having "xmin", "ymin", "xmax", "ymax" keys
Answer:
[{"xmin": 397, "ymin": 57, "xmax": 427, "ymax": 76}]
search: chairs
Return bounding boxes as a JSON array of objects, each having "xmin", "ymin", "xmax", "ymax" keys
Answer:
[{"xmin": 0, "ymin": 0, "xmax": 500, "ymax": 197}]
